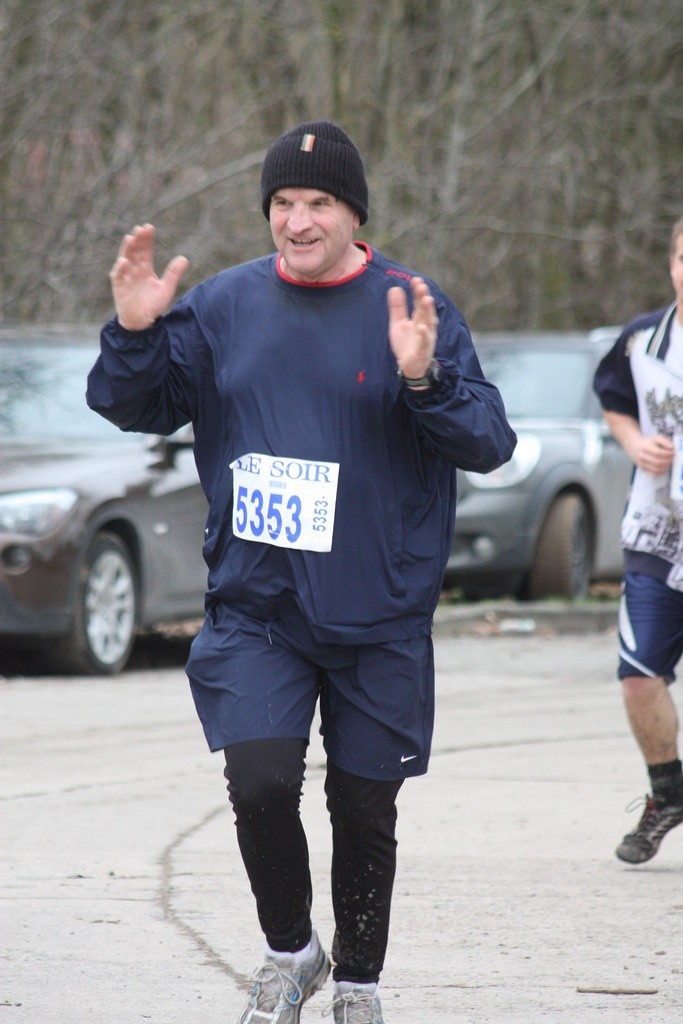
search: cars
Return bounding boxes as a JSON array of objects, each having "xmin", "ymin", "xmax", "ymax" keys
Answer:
[{"xmin": 1, "ymin": 321, "xmax": 215, "ymax": 682}]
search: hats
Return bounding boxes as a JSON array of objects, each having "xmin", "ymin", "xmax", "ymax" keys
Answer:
[{"xmin": 260, "ymin": 120, "xmax": 369, "ymax": 225}]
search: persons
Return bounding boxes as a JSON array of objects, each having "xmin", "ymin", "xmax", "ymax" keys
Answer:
[
  {"xmin": 85, "ymin": 122, "xmax": 516, "ymax": 1024},
  {"xmin": 595, "ymin": 220, "xmax": 683, "ymax": 864}
]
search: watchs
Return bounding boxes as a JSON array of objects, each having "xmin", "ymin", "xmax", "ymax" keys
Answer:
[{"xmin": 396, "ymin": 357, "xmax": 442, "ymax": 387}]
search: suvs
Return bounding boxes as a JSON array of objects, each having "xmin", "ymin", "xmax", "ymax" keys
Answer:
[{"xmin": 441, "ymin": 326, "xmax": 645, "ymax": 602}]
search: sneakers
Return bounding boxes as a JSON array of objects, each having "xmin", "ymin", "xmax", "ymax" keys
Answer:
[
  {"xmin": 612, "ymin": 795, "xmax": 683, "ymax": 863},
  {"xmin": 236, "ymin": 929, "xmax": 331, "ymax": 1024},
  {"xmin": 322, "ymin": 981, "xmax": 385, "ymax": 1024}
]
